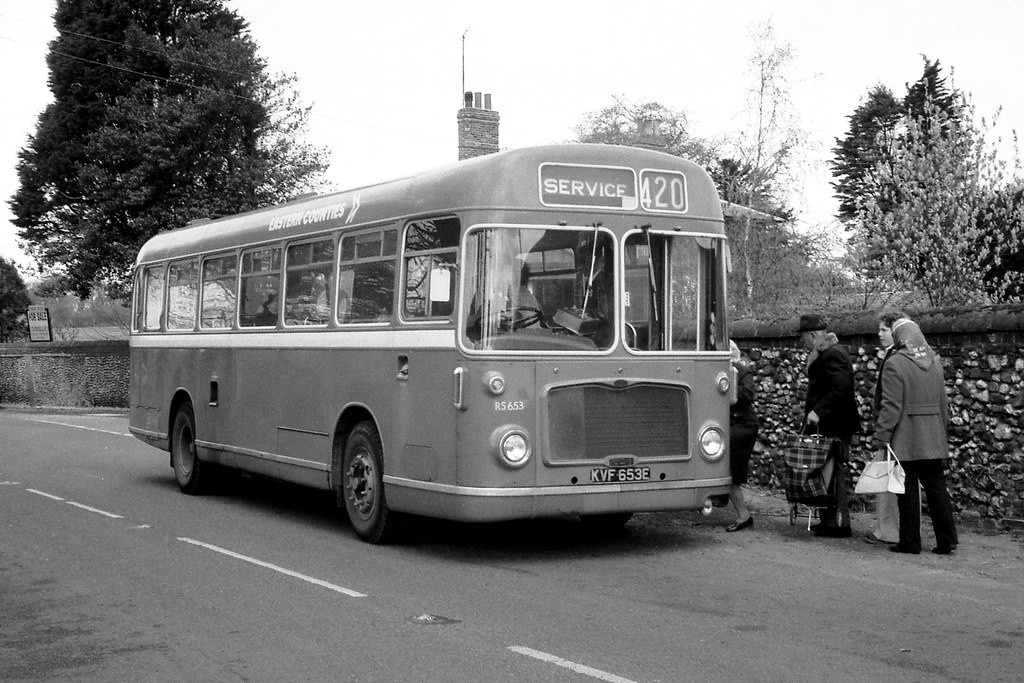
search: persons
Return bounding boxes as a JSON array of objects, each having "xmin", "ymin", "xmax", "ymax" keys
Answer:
[
  {"xmin": 705, "ymin": 339, "xmax": 761, "ymax": 532},
  {"xmin": 470, "ymin": 257, "xmax": 542, "ymax": 328},
  {"xmin": 864, "ymin": 308, "xmax": 912, "ymax": 545},
  {"xmin": 873, "ymin": 318, "xmax": 960, "ymax": 554},
  {"xmin": 791, "ymin": 313, "xmax": 860, "ymax": 538}
]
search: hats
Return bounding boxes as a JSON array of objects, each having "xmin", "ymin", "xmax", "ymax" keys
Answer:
[{"xmin": 795, "ymin": 314, "xmax": 827, "ymax": 333}]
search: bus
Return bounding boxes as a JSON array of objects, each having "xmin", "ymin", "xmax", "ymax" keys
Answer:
[{"xmin": 123, "ymin": 142, "xmax": 740, "ymax": 546}]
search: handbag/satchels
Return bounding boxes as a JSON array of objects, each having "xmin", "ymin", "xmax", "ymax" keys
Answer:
[
  {"xmin": 784, "ymin": 419, "xmax": 841, "ymax": 507},
  {"xmin": 854, "ymin": 442, "xmax": 906, "ymax": 494}
]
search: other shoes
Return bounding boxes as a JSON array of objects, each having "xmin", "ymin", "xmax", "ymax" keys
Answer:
[
  {"xmin": 811, "ymin": 521, "xmax": 824, "ymax": 530},
  {"xmin": 932, "ymin": 540, "xmax": 959, "ymax": 554},
  {"xmin": 885, "ymin": 542, "xmax": 921, "ymax": 555},
  {"xmin": 814, "ymin": 525, "xmax": 853, "ymax": 537},
  {"xmin": 725, "ymin": 515, "xmax": 753, "ymax": 531},
  {"xmin": 864, "ymin": 533, "xmax": 894, "ymax": 545}
]
